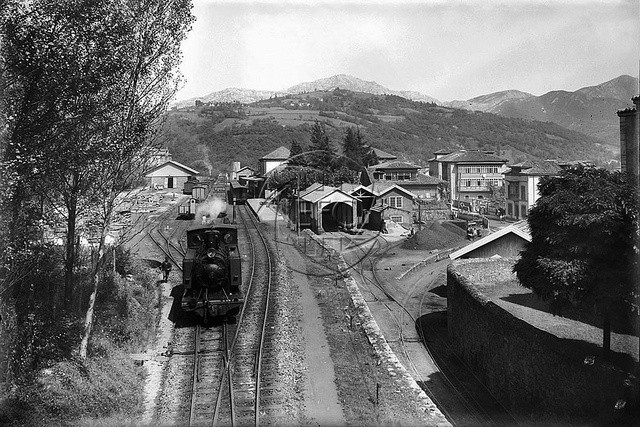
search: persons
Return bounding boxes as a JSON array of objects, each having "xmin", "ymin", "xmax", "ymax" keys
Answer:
[
  {"xmin": 467, "ymin": 222, "xmax": 481, "ymax": 241},
  {"xmin": 500, "ymin": 213, "xmax": 506, "ymax": 224}
]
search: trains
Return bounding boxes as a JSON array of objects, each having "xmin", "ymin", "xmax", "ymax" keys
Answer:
[
  {"xmin": 182, "ymin": 173, "xmax": 246, "ymax": 322},
  {"xmin": 192, "ymin": 173, "xmax": 219, "ymax": 202},
  {"xmin": 227, "ymin": 181, "xmax": 248, "ymax": 205}
]
